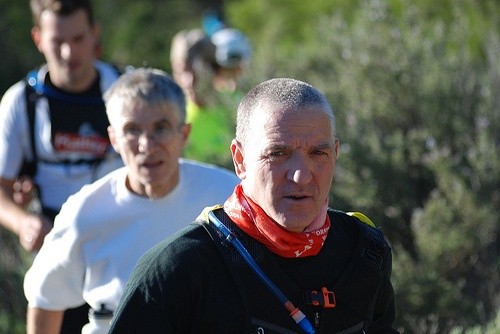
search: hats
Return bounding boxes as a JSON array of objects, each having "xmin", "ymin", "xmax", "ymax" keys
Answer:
[{"xmin": 211, "ymin": 28, "xmax": 252, "ymax": 68}]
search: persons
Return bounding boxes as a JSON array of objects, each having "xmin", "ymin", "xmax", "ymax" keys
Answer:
[
  {"xmin": 0, "ymin": 0, "xmax": 123, "ymax": 333},
  {"xmin": 211, "ymin": 30, "xmax": 253, "ymax": 89},
  {"xmin": 169, "ymin": 29, "xmax": 236, "ymax": 168},
  {"xmin": 22, "ymin": 68, "xmax": 243, "ymax": 332},
  {"xmin": 107, "ymin": 79, "xmax": 395, "ymax": 334}
]
